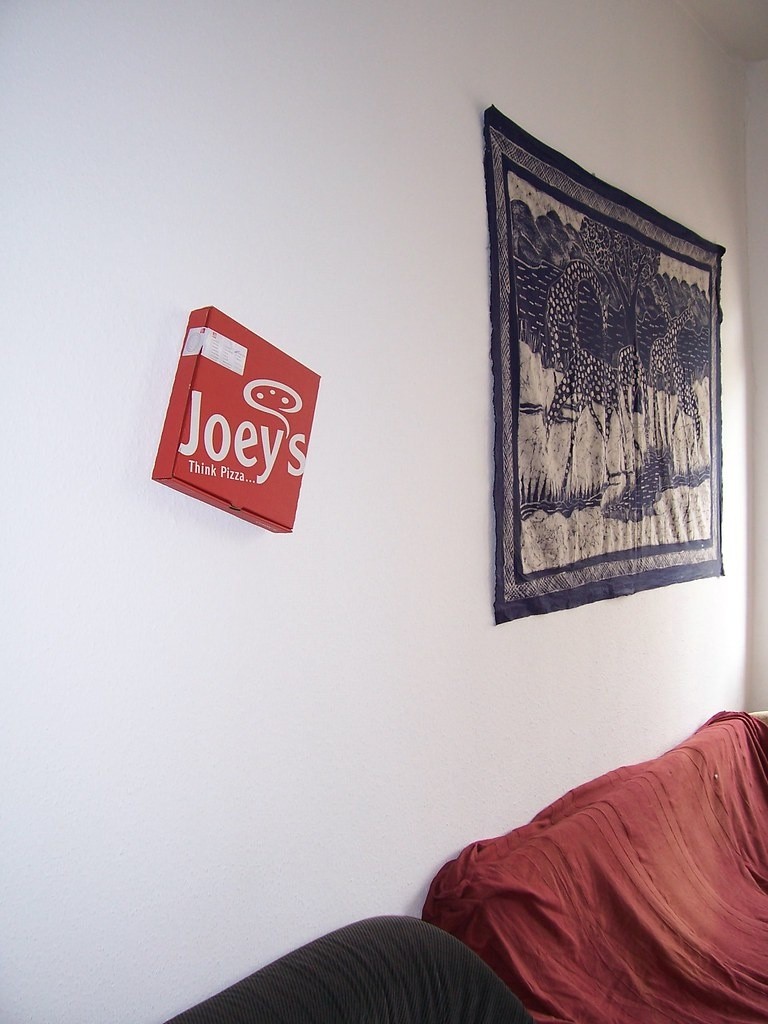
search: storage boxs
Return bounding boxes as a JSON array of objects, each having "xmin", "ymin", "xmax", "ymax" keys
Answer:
[{"xmin": 153, "ymin": 305, "xmax": 324, "ymax": 538}]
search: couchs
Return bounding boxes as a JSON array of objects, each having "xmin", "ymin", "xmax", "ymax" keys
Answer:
[
  {"xmin": 422, "ymin": 710, "xmax": 768, "ymax": 1023},
  {"xmin": 161, "ymin": 916, "xmax": 536, "ymax": 1024}
]
